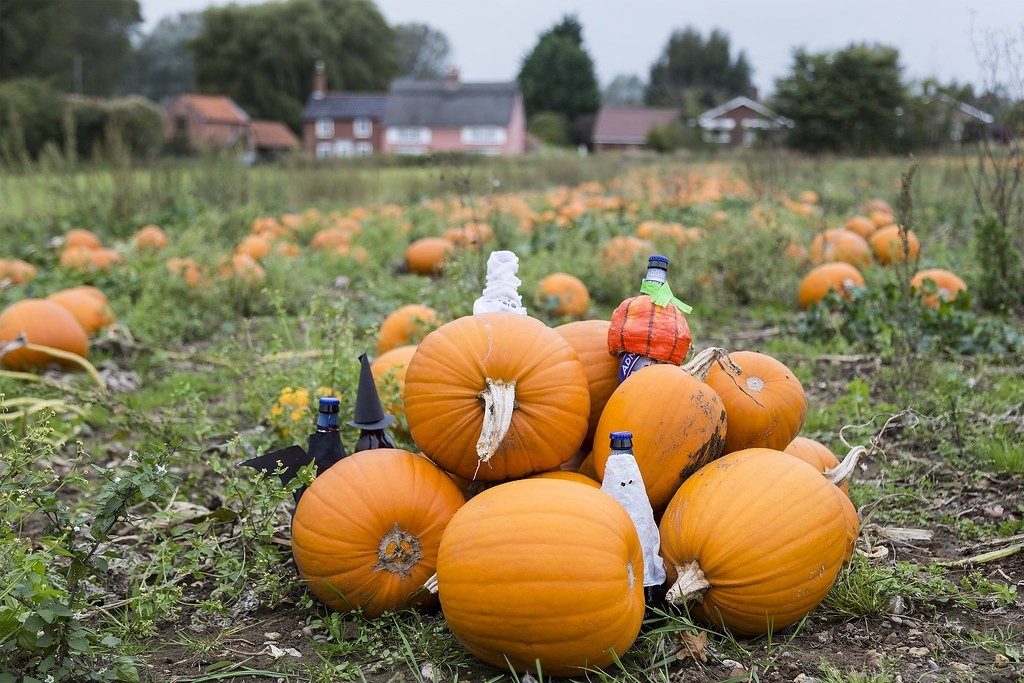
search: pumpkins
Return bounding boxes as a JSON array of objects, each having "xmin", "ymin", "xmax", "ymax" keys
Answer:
[{"xmin": 0, "ymin": 173, "xmax": 968, "ymax": 674}]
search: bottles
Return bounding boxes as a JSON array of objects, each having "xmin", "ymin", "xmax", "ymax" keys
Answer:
[
  {"xmin": 356, "ymin": 428, "xmax": 395, "ymax": 453},
  {"xmin": 619, "ymin": 256, "xmax": 669, "ymax": 385},
  {"xmin": 306, "ymin": 398, "xmax": 339, "ymax": 453},
  {"xmin": 609, "ymin": 432, "xmax": 661, "ymax": 603}
]
